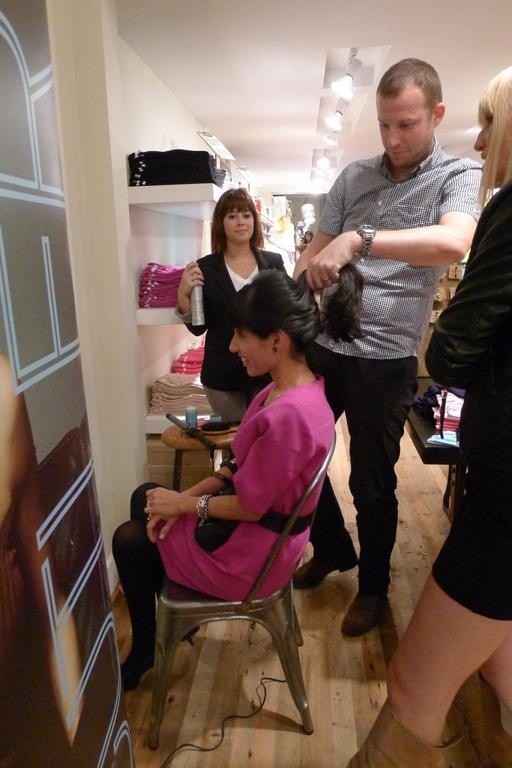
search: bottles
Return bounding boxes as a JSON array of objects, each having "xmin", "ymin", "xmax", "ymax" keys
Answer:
[
  {"xmin": 190, "ymin": 263, "xmax": 205, "ymax": 326},
  {"xmin": 184, "ymin": 405, "xmax": 197, "ymax": 428}
]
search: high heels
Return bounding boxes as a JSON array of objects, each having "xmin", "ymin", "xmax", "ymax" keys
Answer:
[
  {"xmin": 119, "ymin": 652, "xmax": 155, "ymax": 691},
  {"xmin": 181, "ymin": 627, "xmax": 200, "ymax": 647}
]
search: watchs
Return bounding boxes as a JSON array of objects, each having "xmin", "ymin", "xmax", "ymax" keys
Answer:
[{"xmin": 356, "ymin": 223, "xmax": 377, "ymax": 257}]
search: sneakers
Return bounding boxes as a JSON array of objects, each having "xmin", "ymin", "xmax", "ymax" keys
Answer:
[
  {"xmin": 342, "ymin": 580, "xmax": 388, "ymax": 636},
  {"xmin": 293, "ymin": 545, "xmax": 358, "ymax": 587}
]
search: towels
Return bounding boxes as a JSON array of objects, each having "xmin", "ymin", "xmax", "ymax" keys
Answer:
[
  {"xmin": 146, "ymin": 346, "xmax": 212, "ymax": 413},
  {"xmin": 137, "ymin": 261, "xmax": 191, "ymax": 309},
  {"xmin": 127, "ymin": 148, "xmax": 227, "ymax": 188}
]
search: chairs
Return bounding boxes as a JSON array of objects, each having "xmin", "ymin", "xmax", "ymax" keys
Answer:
[{"xmin": 145, "ymin": 428, "xmax": 338, "ymax": 750}]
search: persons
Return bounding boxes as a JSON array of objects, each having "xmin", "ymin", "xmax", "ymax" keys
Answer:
[
  {"xmin": 346, "ymin": 63, "xmax": 511, "ymax": 768},
  {"xmin": 291, "ymin": 56, "xmax": 489, "ymax": 639},
  {"xmin": 296, "ymin": 230, "xmax": 314, "ymax": 255},
  {"xmin": 173, "ymin": 187, "xmax": 289, "ymax": 466},
  {"xmin": 111, "ymin": 261, "xmax": 369, "ymax": 695}
]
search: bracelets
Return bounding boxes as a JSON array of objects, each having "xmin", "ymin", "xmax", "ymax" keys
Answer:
[
  {"xmin": 211, "ymin": 472, "xmax": 229, "ymax": 485},
  {"xmin": 196, "ymin": 494, "xmax": 214, "ymax": 528}
]
